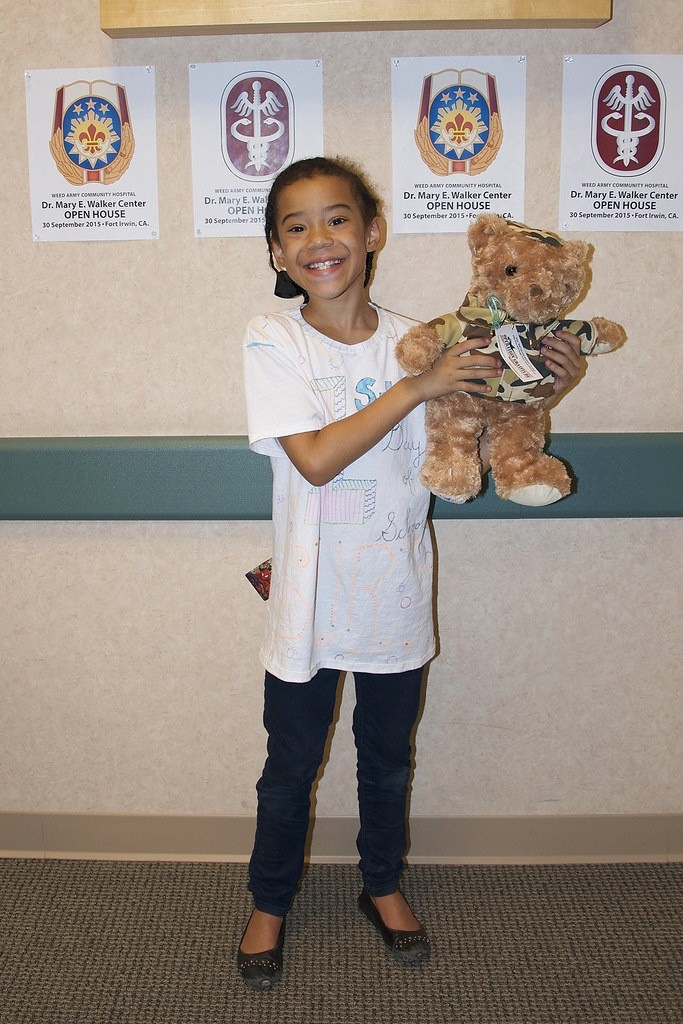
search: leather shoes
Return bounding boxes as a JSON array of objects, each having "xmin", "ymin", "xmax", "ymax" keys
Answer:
[
  {"xmin": 236, "ymin": 905, "xmax": 286, "ymax": 989},
  {"xmin": 356, "ymin": 887, "xmax": 430, "ymax": 965}
]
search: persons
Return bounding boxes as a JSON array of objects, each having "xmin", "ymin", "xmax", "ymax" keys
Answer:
[{"xmin": 236, "ymin": 158, "xmax": 581, "ymax": 990}]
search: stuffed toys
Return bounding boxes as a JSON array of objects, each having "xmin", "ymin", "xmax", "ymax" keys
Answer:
[{"xmin": 394, "ymin": 212, "xmax": 628, "ymax": 507}]
